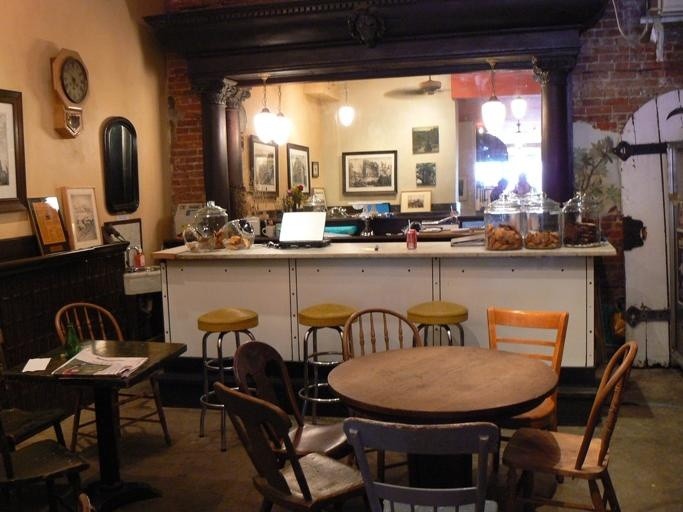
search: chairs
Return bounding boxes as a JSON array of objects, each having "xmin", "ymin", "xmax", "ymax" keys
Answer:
[
  {"xmin": 500, "ymin": 339, "xmax": 638, "ymax": 511},
  {"xmin": 0, "ymin": 414, "xmax": 90, "ymax": 511},
  {"xmin": 54, "ymin": 302, "xmax": 171, "ymax": 453},
  {"xmin": 215, "ymin": 381, "xmax": 366, "ymax": 512},
  {"xmin": 233, "ymin": 340, "xmax": 358, "ymax": 468},
  {"xmin": 486, "ymin": 306, "xmax": 570, "ymax": 484},
  {"xmin": 0, "ymin": 342, "xmax": 75, "ymax": 479},
  {"xmin": 343, "ymin": 415, "xmax": 501, "ymax": 511}
]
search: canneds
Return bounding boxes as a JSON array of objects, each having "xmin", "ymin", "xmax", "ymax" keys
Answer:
[{"xmin": 406, "ymin": 229, "xmax": 417, "ymax": 250}]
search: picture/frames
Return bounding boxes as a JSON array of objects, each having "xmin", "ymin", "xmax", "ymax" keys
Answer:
[
  {"xmin": 249, "ymin": 135, "xmax": 279, "ymax": 199},
  {"xmin": 312, "ymin": 162, "xmax": 319, "ymax": 178},
  {"xmin": 287, "ymin": 143, "xmax": 311, "ymax": 195},
  {"xmin": 103, "ymin": 218, "xmax": 143, "ymax": 268},
  {"xmin": 27, "ymin": 195, "xmax": 69, "ymax": 256},
  {"xmin": 342, "ymin": 150, "xmax": 398, "ymax": 196},
  {"xmin": 61, "ymin": 186, "xmax": 103, "ymax": 250},
  {"xmin": 0, "ymin": 88, "xmax": 29, "ymax": 214},
  {"xmin": 400, "ymin": 190, "xmax": 433, "ymax": 214}
]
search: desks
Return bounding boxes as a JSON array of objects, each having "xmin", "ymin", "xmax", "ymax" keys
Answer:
[
  {"xmin": 327, "ymin": 347, "xmax": 560, "ymax": 506},
  {"xmin": 0, "ymin": 339, "xmax": 187, "ymax": 483}
]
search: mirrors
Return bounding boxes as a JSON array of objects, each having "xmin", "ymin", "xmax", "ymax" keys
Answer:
[{"xmin": 102, "ymin": 116, "xmax": 140, "ymax": 216}]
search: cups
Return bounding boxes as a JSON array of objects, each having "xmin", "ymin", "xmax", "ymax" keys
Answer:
[{"xmin": 407, "ymin": 228, "xmax": 417, "ymax": 249}]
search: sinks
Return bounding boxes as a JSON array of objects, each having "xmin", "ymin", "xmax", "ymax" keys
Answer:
[{"xmin": 122, "ymin": 264, "xmax": 162, "ymax": 295}]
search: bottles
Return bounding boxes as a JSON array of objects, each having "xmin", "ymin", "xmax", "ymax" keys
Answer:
[
  {"xmin": 562, "ymin": 193, "xmax": 601, "ymax": 247},
  {"xmin": 524, "ymin": 192, "xmax": 562, "ymax": 249},
  {"xmin": 195, "ymin": 201, "xmax": 228, "ymax": 239},
  {"xmin": 134, "ymin": 249, "xmax": 146, "ymax": 267},
  {"xmin": 484, "ymin": 193, "xmax": 524, "ymax": 250},
  {"xmin": 65, "ymin": 322, "xmax": 81, "ymax": 356}
]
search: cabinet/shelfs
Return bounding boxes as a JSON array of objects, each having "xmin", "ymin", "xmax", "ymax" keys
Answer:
[{"xmin": 0, "ymin": 240, "xmax": 130, "ymax": 416}]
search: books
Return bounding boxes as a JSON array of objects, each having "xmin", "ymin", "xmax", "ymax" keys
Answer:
[{"xmin": 451, "ymin": 233, "xmax": 485, "ymax": 246}]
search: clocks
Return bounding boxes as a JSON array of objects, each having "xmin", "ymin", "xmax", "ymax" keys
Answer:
[{"xmin": 50, "ymin": 48, "xmax": 91, "ymax": 109}]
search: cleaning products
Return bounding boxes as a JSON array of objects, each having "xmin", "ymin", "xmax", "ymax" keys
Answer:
[{"xmin": 134, "ymin": 248, "xmax": 147, "ymax": 267}]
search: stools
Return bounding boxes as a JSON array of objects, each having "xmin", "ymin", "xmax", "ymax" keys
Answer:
[
  {"xmin": 299, "ymin": 302, "xmax": 359, "ymax": 425},
  {"xmin": 407, "ymin": 301, "xmax": 469, "ymax": 347},
  {"xmin": 198, "ymin": 307, "xmax": 259, "ymax": 452}
]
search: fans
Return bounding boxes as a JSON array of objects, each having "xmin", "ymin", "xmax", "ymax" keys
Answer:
[{"xmin": 385, "ymin": 75, "xmax": 475, "ymax": 97}]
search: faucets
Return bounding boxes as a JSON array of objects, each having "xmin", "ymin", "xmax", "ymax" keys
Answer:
[{"xmin": 125, "ymin": 245, "xmax": 143, "ymax": 267}]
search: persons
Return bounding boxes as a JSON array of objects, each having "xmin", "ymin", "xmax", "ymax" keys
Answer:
[{"xmin": 62, "ymin": 364, "xmax": 81, "ymax": 376}]
